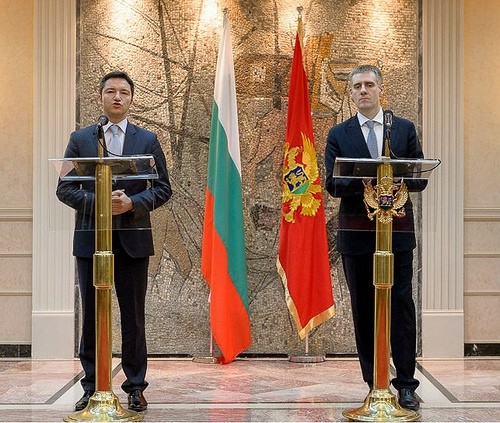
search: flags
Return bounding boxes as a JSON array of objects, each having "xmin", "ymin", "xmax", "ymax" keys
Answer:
[
  {"xmin": 274, "ymin": 13, "xmax": 336, "ymax": 340},
  {"xmin": 198, "ymin": 10, "xmax": 253, "ymax": 367}
]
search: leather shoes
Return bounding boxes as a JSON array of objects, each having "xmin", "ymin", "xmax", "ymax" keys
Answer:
[
  {"xmin": 75, "ymin": 391, "xmax": 92, "ymax": 410},
  {"xmin": 398, "ymin": 388, "xmax": 420, "ymax": 412},
  {"xmin": 128, "ymin": 390, "xmax": 147, "ymax": 411}
]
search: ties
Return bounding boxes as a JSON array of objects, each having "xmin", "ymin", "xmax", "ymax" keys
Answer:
[
  {"xmin": 108, "ymin": 126, "xmax": 122, "ymax": 159},
  {"xmin": 366, "ymin": 121, "xmax": 378, "ymax": 159}
]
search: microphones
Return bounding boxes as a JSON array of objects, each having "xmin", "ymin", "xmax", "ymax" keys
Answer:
[
  {"xmin": 383, "ymin": 110, "xmax": 393, "ymax": 128},
  {"xmin": 97, "ymin": 114, "xmax": 110, "ymax": 127}
]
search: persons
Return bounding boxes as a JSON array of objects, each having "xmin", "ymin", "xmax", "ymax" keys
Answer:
[
  {"xmin": 323, "ymin": 66, "xmax": 427, "ymax": 410},
  {"xmin": 56, "ymin": 72, "xmax": 173, "ymax": 411}
]
